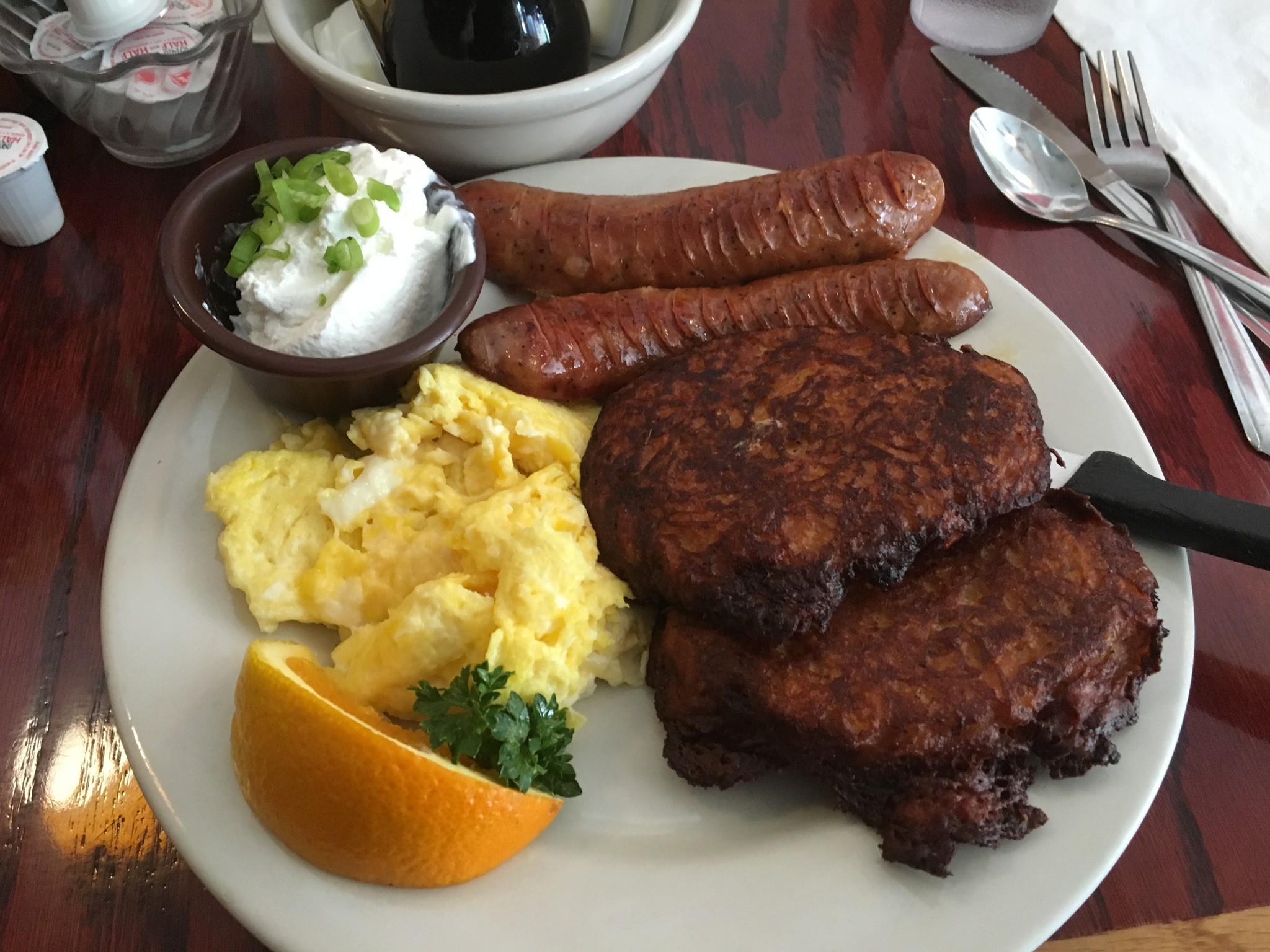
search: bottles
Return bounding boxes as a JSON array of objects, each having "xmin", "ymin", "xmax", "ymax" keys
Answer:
[{"xmin": 354, "ymin": 0, "xmax": 591, "ymax": 97}]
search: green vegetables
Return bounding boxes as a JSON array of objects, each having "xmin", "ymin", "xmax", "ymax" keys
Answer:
[{"xmin": 405, "ymin": 660, "xmax": 583, "ymax": 798}]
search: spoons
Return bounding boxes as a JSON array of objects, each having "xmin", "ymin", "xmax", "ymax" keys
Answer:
[{"xmin": 969, "ymin": 106, "xmax": 1270, "ymax": 307}]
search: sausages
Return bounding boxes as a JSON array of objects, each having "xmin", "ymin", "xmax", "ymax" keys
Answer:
[
  {"xmin": 455, "ymin": 149, "xmax": 946, "ymax": 301},
  {"xmin": 454, "ymin": 259, "xmax": 992, "ymax": 405}
]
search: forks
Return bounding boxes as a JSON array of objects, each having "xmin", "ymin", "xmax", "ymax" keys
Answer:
[{"xmin": 1080, "ymin": 51, "xmax": 1270, "ymax": 454}]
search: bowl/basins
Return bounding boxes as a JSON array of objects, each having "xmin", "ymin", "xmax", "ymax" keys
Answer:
[{"xmin": 263, "ymin": 0, "xmax": 704, "ymax": 183}]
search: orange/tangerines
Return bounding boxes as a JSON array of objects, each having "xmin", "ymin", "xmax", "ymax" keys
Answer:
[{"xmin": 229, "ymin": 638, "xmax": 567, "ymax": 890}]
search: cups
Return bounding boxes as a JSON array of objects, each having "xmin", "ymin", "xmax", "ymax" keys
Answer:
[
  {"xmin": 0, "ymin": 113, "xmax": 65, "ymax": 248},
  {"xmin": 0, "ymin": 0, "xmax": 263, "ymax": 167},
  {"xmin": 162, "ymin": 136, "xmax": 486, "ymax": 415},
  {"xmin": 30, "ymin": 0, "xmax": 224, "ymax": 146}
]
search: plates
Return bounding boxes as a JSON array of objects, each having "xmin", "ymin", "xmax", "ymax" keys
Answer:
[{"xmin": 98, "ymin": 158, "xmax": 1196, "ymax": 952}]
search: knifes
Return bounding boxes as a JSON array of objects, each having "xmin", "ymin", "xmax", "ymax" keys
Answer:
[
  {"xmin": 1050, "ymin": 446, "xmax": 1270, "ymax": 574},
  {"xmin": 931, "ymin": 46, "xmax": 1270, "ymax": 349}
]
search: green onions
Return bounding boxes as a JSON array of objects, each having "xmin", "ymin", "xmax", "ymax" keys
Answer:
[{"xmin": 223, "ymin": 148, "xmax": 399, "ymax": 308}]
